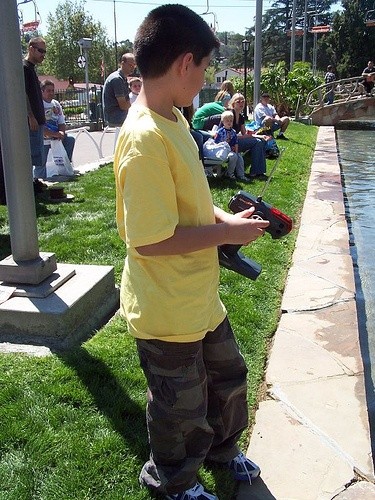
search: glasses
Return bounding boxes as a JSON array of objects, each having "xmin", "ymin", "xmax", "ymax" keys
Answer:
[
  {"xmin": 234, "ymin": 99, "xmax": 244, "ymax": 104},
  {"xmin": 262, "ymin": 95, "xmax": 270, "ymax": 98},
  {"xmin": 126, "ymin": 62, "xmax": 136, "ymax": 68},
  {"xmin": 34, "ymin": 46, "xmax": 46, "ymax": 54}
]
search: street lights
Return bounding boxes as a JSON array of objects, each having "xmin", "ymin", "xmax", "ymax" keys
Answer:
[{"xmin": 241, "ymin": 38, "xmax": 252, "ymax": 120}]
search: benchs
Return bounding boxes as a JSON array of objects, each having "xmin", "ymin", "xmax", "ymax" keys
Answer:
[
  {"xmin": 204, "ymin": 134, "xmax": 270, "ymax": 176},
  {"xmin": 65, "ymin": 124, "xmax": 121, "ymax": 167}
]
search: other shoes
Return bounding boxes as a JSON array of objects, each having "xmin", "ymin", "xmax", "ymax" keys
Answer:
[
  {"xmin": 276, "ymin": 134, "xmax": 289, "ymax": 140},
  {"xmin": 253, "ymin": 174, "xmax": 268, "ymax": 180},
  {"xmin": 237, "ymin": 176, "xmax": 250, "ymax": 181},
  {"xmin": 225, "ymin": 172, "xmax": 236, "ymax": 180}
]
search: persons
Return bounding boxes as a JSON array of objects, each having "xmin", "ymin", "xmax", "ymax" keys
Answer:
[
  {"xmin": 20, "ymin": 36, "xmax": 49, "ymax": 189},
  {"xmin": 127, "ymin": 77, "xmax": 143, "ymax": 106},
  {"xmin": 101, "ymin": 52, "xmax": 137, "ymax": 127},
  {"xmin": 357, "ymin": 60, "xmax": 375, "ymax": 97},
  {"xmin": 174, "ymin": 79, "xmax": 290, "ymax": 184},
  {"xmin": 324, "ymin": 64, "xmax": 335, "ymax": 105},
  {"xmin": 112, "ymin": 3, "xmax": 277, "ymax": 500},
  {"xmin": 33, "ymin": 78, "xmax": 76, "ymax": 179}
]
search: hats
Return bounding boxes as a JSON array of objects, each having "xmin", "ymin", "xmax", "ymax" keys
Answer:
[{"xmin": 40, "ymin": 186, "xmax": 75, "ymax": 203}]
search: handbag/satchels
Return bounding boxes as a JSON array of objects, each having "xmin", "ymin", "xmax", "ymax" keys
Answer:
[
  {"xmin": 202, "ymin": 138, "xmax": 230, "ymax": 161},
  {"xmin": 46, "ymin": 139, "xmax": 74, "ymax": 179}
]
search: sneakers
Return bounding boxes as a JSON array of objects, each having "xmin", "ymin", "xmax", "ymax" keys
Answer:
[
  {"xmin": 205, "ymin": 452, "xmax": 261, "ymax": 486},
  {"xmin": 163, "ymin": 482, "xmax": 218, "ymax": 500}
]
